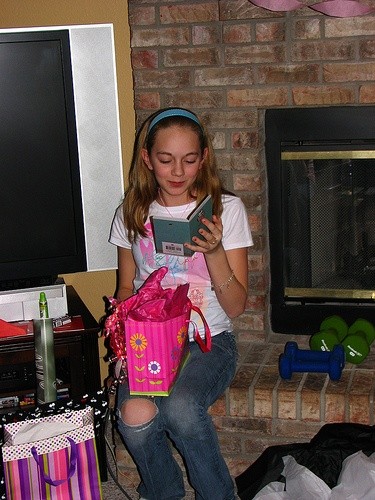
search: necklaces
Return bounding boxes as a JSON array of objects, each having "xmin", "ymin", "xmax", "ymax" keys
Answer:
[{"xmin": 157, "ymin": 186, "xmax": 199, "ymax": 222}]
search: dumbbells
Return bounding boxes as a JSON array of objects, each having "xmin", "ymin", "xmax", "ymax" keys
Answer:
[
  {"xmin": 280, "ymin": 352, "xmax": 340, "ymax": 381},
  {"xmin": 284, "ymin": 341, "xmax": 345, "ymax": 369},
  {"xmin": 309, "ymin": 315, "xmax": 348, "ymax": 350},
  {"xmin": 340, "ymin": 318, "xmax": 375, "ymax": 364}
]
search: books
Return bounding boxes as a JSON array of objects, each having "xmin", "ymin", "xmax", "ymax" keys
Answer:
[{"xmin": 152, "ymin": 195, "xmax": 215, "ymax": 258}]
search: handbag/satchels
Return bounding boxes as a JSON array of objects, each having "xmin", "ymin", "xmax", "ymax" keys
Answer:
[
  {"xmin": 125, "ymin": 288, "xmax": 212, "ymax": 397},
  {"xmin": 1, "ymin": 407, "xmax": 103, "ymax": 500}
]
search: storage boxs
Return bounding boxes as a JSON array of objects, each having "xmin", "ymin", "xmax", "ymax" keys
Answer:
[{"xmin": 0, "ymin": 284, "xmax": 68, "ymax": 322}]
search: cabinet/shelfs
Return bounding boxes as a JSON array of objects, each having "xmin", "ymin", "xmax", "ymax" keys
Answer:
[{"xmin": 0, "ymin": 285, "xmax": 108, "ymax": 483}]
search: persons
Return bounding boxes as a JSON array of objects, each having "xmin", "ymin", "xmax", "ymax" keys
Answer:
[{"xmin": 104, "ymin": 106, "xmax": 254, "ymax": 500}]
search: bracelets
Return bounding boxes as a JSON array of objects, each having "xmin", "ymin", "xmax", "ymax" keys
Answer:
[{"xmin": 209, "ymin": 268, "xmax": 235, "ymax": 294}]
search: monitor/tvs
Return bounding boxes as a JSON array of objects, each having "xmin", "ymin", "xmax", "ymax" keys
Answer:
[{"xmin": 0, "ymin": 23, "xmax": 125, "ymax": 289}]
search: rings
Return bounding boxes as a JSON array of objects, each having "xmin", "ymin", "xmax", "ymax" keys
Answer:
[{"xmin": 210, "ymin": 238, "xmax": 217, "ymax": 244}]
